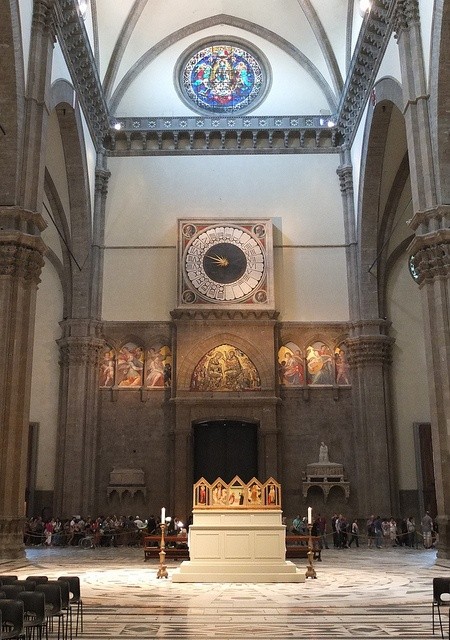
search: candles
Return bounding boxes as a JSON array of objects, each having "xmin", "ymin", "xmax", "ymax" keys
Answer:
[
  {"xmin": 307, "ymin": 506, "xmax": 313, "ymax": 525},
  {"xmin": 161, "ymin": 507, "xmax": 166, "ymax": 524}
]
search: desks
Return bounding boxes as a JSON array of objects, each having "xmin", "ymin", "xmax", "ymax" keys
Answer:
[
  {"xmin": 286, "ymin": 534, "xmax": 323, "ymax": 562},
  {"xmin": 143, "ymin": 533, "xmax": 190, "ymax": 561}
]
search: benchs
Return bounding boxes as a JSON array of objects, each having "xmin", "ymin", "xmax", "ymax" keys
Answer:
[
  {"xmin": 286, "ymin": 545, "xmax": 322, "ymax": 559},
  {"xmin": 145, "ymin": 548, "xmax": 189, "ymax": 559}
]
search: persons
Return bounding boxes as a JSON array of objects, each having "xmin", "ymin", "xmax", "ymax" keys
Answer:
[
  {"xmin": 125, "ymin": 353, "xmax": 133, "ymax": 381},
  {"xmin": 26, "ymin": 514, "xmax": 192, "ymax": 554},
  {"xmin": 120, "ymin": 346, "xmax": 132, "ymax": 381},
  {"xmin": 148, "ymin": 346, "xmax": 170, "ymax": 388},
  {"xmin": 332, "ymin": 346, "xmax": 347, "ymax": 388},
  {"xmin": 133, "ymin": 348, "xmax": 143, "ymax": 377},
  {"xmin": 101, "ymin": 350, "xmax": 117, "ymax": 386},
  {"xmin": 225, "ymin": 350, "xmax": 243, "ymax": 391},
  {"xmin": 206, "ymin": 350, "xmax": 223, "ymax": 390},
  {"xmin": 288, "ymin": 512, "xmax": 439, "ymax": 552},
  {"xmin": 294, "ymin": 350, "xmax": 302, "ymax": 384},
  {"xmin": 284, "ymin": 351, "xmax": 292, "ymax": 384},
  {"xmin": 308, "ymin": 346, "xmax": 332, "ymax": 385}
]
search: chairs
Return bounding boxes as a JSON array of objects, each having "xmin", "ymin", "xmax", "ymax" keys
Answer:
[
  {"xmin": 431, "ymin": 576, "xmax": 450, "ymax": 639},
  {"xmin": 0, "ymin": 576, "xmax": 83, "ymax": 639}
]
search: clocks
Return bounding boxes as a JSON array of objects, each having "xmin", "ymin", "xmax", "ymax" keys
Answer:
[{"xmin": 173, "ymin": 216, "xmax": 276, "ymax": 314}]
